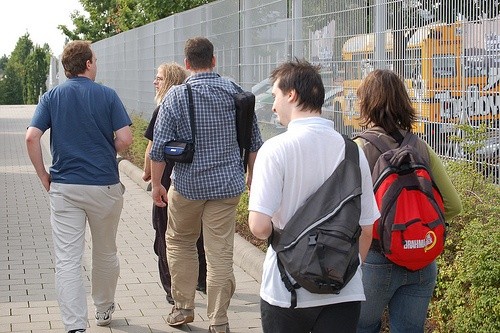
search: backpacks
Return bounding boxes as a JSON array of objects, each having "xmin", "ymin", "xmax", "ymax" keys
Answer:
[{"xmin": 351, "ymin": 131, "xmax": 449, "ymax": 273}]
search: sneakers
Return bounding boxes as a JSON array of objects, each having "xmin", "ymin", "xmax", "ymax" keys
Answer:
[
  {"xmin": 166, "ymin": 305, "xmax": 196, "ymax": 326},
  {"xmin": 95, "ymin": 300, "xmax": 116, "ymax": 325},
  {"xmin": 208, "ymin": 322, "xmax": 230, "ymax": 333}
]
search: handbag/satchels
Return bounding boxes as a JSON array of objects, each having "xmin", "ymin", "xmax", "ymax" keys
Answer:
[
  {"xmin": 165, "ymin": 140, "xmax": 195, "ymax": 164},
  {"xmin": 234, "ymin": 90, "xmax": 257, "ymax": 173},
  {"xmin": 268, "ymin": 133, "xmax": 362, "ymax": 294}
]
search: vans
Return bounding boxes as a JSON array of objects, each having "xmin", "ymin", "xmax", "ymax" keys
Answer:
[{"xmin": 335, "ymin": 24, "xmax": 499, "ymax": 137}]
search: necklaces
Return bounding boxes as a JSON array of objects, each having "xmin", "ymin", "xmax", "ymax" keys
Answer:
[{"xmin": 142, "ymin": 63, "xmax": 207, "ymax": 305}]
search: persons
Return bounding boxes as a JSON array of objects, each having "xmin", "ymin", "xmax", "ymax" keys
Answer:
[
  {"xmin": 25, "ymin": 40, "xmax": 133, "ymax": 333},
  {"xmin": 247, "ymin": 61, "xmax": 381, "ymax": 333},
  {"xmin": 149, "ymin": 36, "xmax": 264, "ymax": 333},
  {"xmin": 351, "ymin": 69, "xmax": 462, "ymax": 333}
]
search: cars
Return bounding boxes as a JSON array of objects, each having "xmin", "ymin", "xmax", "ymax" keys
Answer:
[{"xmin": 246, "ymin": 67, "xmax": 344, "ymax": 139}]
